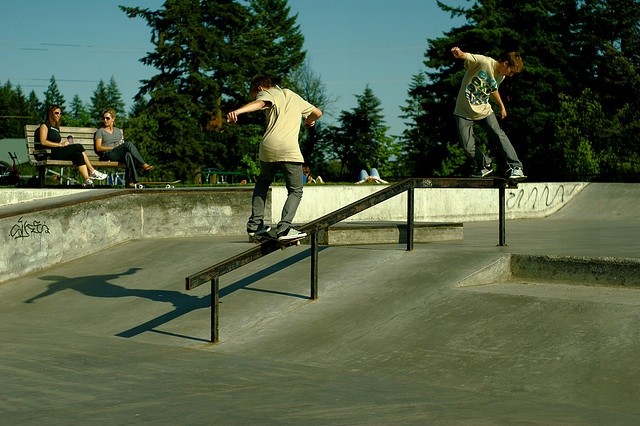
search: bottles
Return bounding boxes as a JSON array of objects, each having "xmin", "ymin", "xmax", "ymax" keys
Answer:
[{"xmin": 67, "ymin": 135, "xmax": 73, "ymax": 144}]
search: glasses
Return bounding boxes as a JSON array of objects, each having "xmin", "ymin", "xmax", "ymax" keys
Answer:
[
  {"xmin": 104, "ymin": 117, "xmax": 111, "ymax": 120},
  {"xmin": 55, "ymin": 112, "xmax": 62, "ymax": 114}
]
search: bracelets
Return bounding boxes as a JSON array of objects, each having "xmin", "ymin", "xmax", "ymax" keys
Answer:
[{"xmin": 304, "ymin": 119, "xmax": 313, "ymax": 124}]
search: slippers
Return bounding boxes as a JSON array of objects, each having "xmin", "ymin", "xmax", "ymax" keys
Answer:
[{"xmin": 143, "ymin": 165, "xmax": 155, "ymax": 173}]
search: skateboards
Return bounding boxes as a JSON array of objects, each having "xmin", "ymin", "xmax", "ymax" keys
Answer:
[
  {"xmin": 471, "ymin": 176, "xmax": 527, "ymax": 186},
  {"xmin": 249, "ymin": 226, "xmax": 305, "ymax": 246},
  {"xmin": 130, "ymin": 180, "xmax": 181, "ymax": 188}
]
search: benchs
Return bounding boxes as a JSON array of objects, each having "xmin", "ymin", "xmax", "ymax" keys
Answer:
[{"xmin": 24, "ymin": 124, "xmax": 126, "ymax": 186}]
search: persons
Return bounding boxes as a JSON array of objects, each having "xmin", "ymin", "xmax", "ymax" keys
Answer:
[
  {"xmin": 299, "ymin": 163, "xmax": 317, "ymax": 185},
  {"xmin": 33, "ymin": 103, "xmax": 108, "ymax": 187},
  {"xmin": 93, "ymin": 107, "xmax": 153, "ymax": 188},
  {"xmin": 226, "ymin": 73, "xmax": 324, "ymax": 243},
  {"xmin": 450, "ymin": 44, "xmax": 528, "ymax": 179},
  {"xmin": 354, "ymin": 166, "xmax": 391, "ymax": 185}
]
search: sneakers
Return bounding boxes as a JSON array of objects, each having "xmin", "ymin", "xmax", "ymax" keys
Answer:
[
  {"xmin": 278, "ymin": 227, "xmax": 308, "ymax": 242},
  {"xmin": 89, "ymin": 170, "xmax": 108, "ymax": 180},
  {"xmin": 248, "ymin": 225, "xmax": 272, "ymax": 236},
  {"xmin": 472, "ymin": 163, "xmax": 494, "ymax": 178},
  {"xmin": 82, "ymin": 180, "xmax": 95, "ymax": 188},
  {"xmin": 506, "ymin": 165, "xmax": 525, "ymax": 179}
]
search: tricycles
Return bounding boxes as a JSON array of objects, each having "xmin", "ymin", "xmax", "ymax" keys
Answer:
[{"xmin": 0, "ymin": 152, "xmax": 21, "ymax": 187}]
